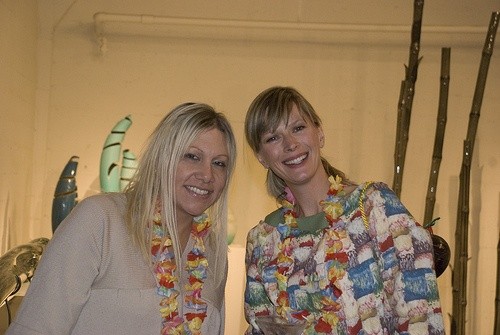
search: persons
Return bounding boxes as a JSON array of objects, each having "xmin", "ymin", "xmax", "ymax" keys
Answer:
[
  {"xmin": 239, "ymin": 86, "xmax": 447, "ymax": 335},
  {"xmin": 4, "ymin": 102, "xmax": 237, "ymax": 335}
]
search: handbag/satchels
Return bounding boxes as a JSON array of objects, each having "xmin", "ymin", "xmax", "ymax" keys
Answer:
[{"xmin": 424, "ymin": 217, "xmax": 451, "ymax": 279}]
